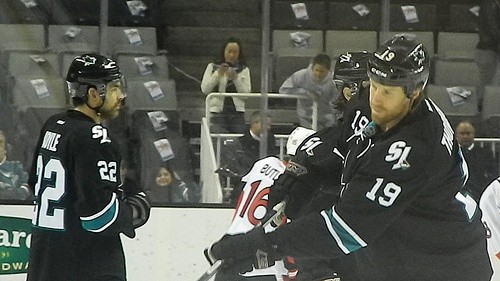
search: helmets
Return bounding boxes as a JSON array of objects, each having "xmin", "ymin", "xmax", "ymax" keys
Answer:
[
  {"xmin": 366, "ymin": 34, "xmax": 430, "ymax": 95},
  {"xmin": 332, "ymin": 51, "xmax": 373, "ymax": 89},
  {"xmin": 286, "ymin": 127, "xmax": 317, "ymax": 155},
  {"xmin": 65, "ymin": 53, "xmax": 121, "ymax": 113}
]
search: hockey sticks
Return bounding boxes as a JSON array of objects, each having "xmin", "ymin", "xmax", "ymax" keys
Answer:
[{"xmin": 198, "ymin": 200, "xmax": 286, "ymax": 281}]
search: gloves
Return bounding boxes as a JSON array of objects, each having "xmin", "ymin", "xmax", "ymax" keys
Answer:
[
  {"xmin": 124, "ymin": 191, "xmax": 151, "ymax": 229},
  {"xmin": 209, "ymin": 227, "xmax": 266, "ymax": 270},
  {"xmin": 266, "ymin": 174, "xmax": 301, "ymax": 227}
]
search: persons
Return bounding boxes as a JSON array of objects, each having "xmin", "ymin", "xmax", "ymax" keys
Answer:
[
  {"xmin": 143, "ymin": 164, "xmax": 190, "ymax": 203},
  {"xmin": 456, "ymin": 121, "xmax": 500, "ymax": 204},
  {"xmin": 25, "ymin": 52, "xmax": 151, "ymax": 281},
  {"xmin": 0, "ymin": 130, "xmax": 31, "ymax": 200},
  {"xmin": 200, "ymin": 37, "xmax": 373, "ymax": 281},
  {"xmin": 478, "ymin": 175, "xmax": 500, "ymax": 281},
  {"xmin": 212, "ymin": 34, "xmax": 493, "ymax": 280}
]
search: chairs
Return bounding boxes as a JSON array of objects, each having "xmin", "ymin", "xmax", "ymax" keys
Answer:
[{"xmin": 0, "ymin": 23, "xmax": 500, "ymax": 137}]
